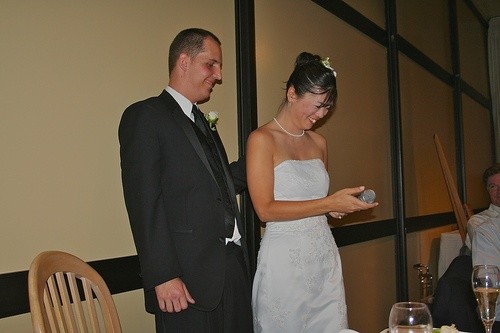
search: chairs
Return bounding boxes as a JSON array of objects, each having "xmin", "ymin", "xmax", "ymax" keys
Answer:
[{"xmin": 28, "ymin": 251, "xmax": 123, "ymax": 333}]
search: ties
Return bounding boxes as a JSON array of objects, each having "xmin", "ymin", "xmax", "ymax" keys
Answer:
[{"xmin": 191, "ymin": 104, "xmax": 212, "ymax": 137}]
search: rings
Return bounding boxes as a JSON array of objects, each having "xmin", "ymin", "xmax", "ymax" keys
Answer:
[{"xmin": 338, "ymin": 216, "xmax": 342, "ymax": 219}]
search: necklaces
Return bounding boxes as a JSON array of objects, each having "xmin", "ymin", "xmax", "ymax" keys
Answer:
[{"xmin": 273, "ymin": 117, "xmax": 305, "ymax": 137}]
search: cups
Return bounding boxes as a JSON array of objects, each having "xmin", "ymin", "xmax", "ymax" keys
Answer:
[{"xmin": 388, "ymin": 301, "xmax": 433, "ymax": 333}]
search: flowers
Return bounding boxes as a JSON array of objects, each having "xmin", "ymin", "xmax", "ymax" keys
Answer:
[{"xmin": 206, "ymin": 111, "xmax": 219, "ymax": 131}]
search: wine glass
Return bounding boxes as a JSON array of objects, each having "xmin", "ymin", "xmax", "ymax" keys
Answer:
[{"xmin": 471, "ymin": 265, "xmax": 500, "ymax": 333}]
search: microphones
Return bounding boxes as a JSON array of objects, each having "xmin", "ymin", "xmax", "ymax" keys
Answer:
[{"xmin": 355, "ymin": 189, "xmax": 376, "ymax": 204}]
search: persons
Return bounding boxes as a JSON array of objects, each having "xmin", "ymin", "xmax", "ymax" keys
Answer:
[
  {"xmin": 117, "ymin": 28, "xmax": 256, "ymax": 332},
  {"xmin": 461, "ymin": 165, "xmax": 499, "ymax": 333},
  {"xmin": 245, "ymin": 51, "xmax": 377, "ymax": 333}
]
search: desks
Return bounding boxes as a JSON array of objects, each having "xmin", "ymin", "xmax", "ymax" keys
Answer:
[{"xmin": 379, "ymin": 326, "xmax": 464, "ymax": 333}]
